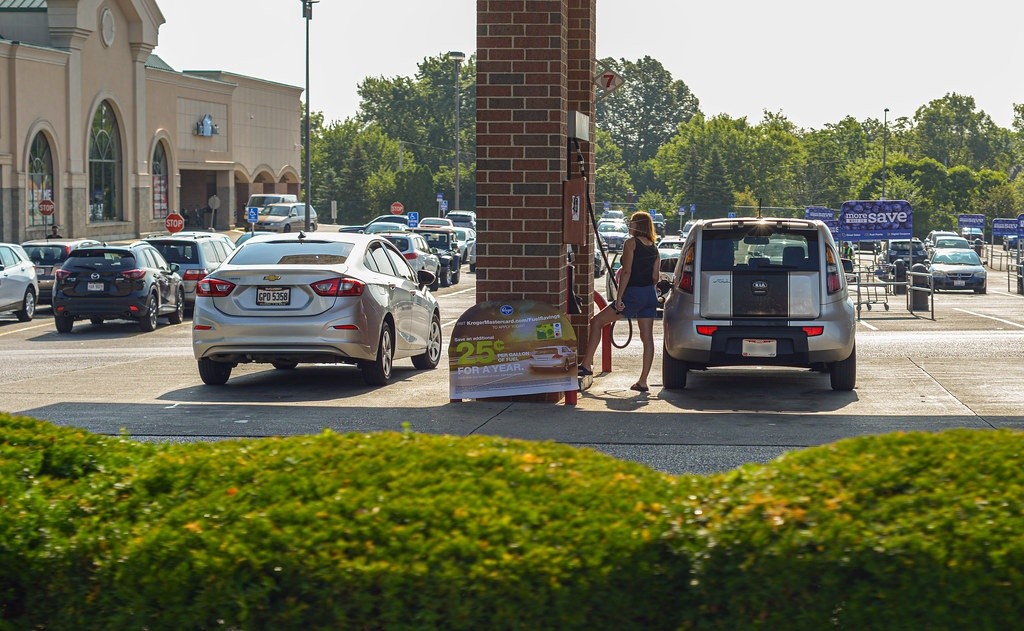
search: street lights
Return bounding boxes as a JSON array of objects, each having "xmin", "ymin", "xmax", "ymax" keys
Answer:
[
  {"xmin": 298, "ymin": 0, "xmax": 321, "ymax": 232},
  {"xmin": 880, "ymin": 107, "xmax": 890, "ymax": 200},
  {"xmin": 448, "ymin": 52, "xmax": 467, "ymax": 211}
]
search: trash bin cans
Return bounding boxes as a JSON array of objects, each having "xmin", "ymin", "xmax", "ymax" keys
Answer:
[
  {"xmin": 893, "ymin": 259, "xmax": 907, "ymax": 295},
  {"xmin": 911, "ymin": 263, "xmax": 929, "ymax": 311},
  {"xmin": 974, "ymin": 239, "xmax": 981, "ymax": 257},
  {"xmin": 1019, "ymin": 261, "xmax": 1024, "ymax": 295}
]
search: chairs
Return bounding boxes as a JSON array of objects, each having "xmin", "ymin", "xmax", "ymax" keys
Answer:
[
  {"xmin": 166, "ymin": 248, "xmax": 180, "ymax": 263},
  {"xmin": 783, "ymin": 247, "xmax": 806, "ymax": 267},
  {"xmin": 31, "ymin": 250, "xmax": 42, "ymax": 260},
  {"xmin": 962, "ymin": 256, "xmax": 971, "ymax": 263},
  {"xmin": 44, "ymin": 249, "xmax": 54, "ymax": 260},
  {"xmin": 120, "ymin": 255, "xmax": 135, "ymax": 267}
]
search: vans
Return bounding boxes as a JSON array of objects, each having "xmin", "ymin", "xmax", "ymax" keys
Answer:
[{"xmin": 242, "ymin": 193, "xmax": 298, "ymax": 231}]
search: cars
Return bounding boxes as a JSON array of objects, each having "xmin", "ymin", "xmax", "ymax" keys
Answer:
[
  {"xmin": 746, "ymin": 239, "xmax": 809, "ymax": 266},
  {"xmin": 338, "ymin": 210, "xmax": 476, "ymax": 291},
  {"xmin": 528, "ymin": 345, "xmax": 578, "ymax": 372},
  {"xmin": 191, "ymin": 229, "xmax": 444, "ymax": 387},
  {"xmin": 594, "ymin": 210, "xmax": 696, "ymax": 314},
  {"xmin": 234, "ymin": 232, "xmax": 278, "ymax": 248},
  {"xmin": 961, "ymin": 227, "xmax": 985, "ymax": 245},
  {"xmin": 877, "ymin": 229, "xmax": 988, "ymax": 294},
  {"xmin": 1002, "ymin": 235, "xmax": 1022, "ymax": 251}
]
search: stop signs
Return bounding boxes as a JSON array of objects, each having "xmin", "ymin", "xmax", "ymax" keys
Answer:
[
  {"xmin": 164, "ymin": 213, "xmax": 185, "ymax": 234},
  {"xmin": 38, "ymin": 200, "xmax": 55, "ymax": 216},
  {"xmin": 390, "ymin": 201, "xmax": 404, "ymax": 215}
]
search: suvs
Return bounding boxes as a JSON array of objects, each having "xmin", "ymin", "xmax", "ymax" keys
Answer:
[
  {"xmin": 19, "ymin": 238, "xmax": 115, "ymax": 306},
  {"xmin": 0, "ymin": 243, "xmax": 40, "ymax": 323},
  {"xmin": 140, "ymin": 231, "xmax": 237, "ymax": 309},
  {"xmin": 658, "ymin": 218, "xmax": 857, "ymax": 392},
  {"xmin": 51, "ymin": 240, "xmax": 185, "ymax": 334},
  {"xmin": 253, "ymin": 203, "xmax": 319, "ymax": 233}
]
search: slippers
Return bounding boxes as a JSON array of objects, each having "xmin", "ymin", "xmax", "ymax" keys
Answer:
[
  {"xmin": 630, "ymin": 383, "xmax": 649, "ymax": 391},
  {"xmin": 578, "ymin": 364, "xmax": 593, "ymax": 375}
]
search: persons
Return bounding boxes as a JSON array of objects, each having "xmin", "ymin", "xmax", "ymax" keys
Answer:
[
  {"xmin": 577, "ymin": 212, "xmax": 662, "ymax": 392},
  {"xmin": 974, "ymin": 232, "xmax": 987, "ymax": 257},
  {"xmin": 239, "ymin": 202, "xmax": 251, "ymax": 232},
  {"xmin": 45, "ymin": 224, "xmax": 63, "ymax": 241},
  {"xmin": 840, "ymin": 241, "xmax": 855, "ymax": 267}
]
